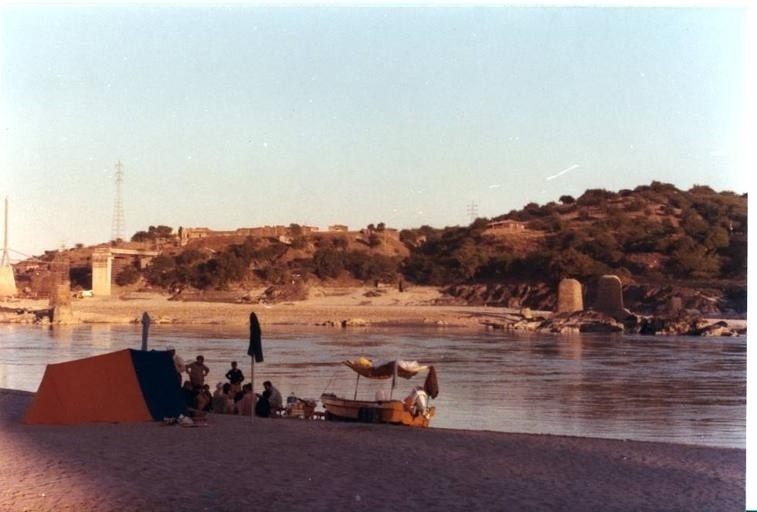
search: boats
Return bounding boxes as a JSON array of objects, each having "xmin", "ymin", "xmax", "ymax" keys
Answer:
[{"xmin": 315, "ymin": 356, "xmax": 438, "ymax": 427}]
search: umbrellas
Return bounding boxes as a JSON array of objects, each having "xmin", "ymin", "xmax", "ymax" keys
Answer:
[
  {"xmin": 422, "ymin": 366, "xmax": 438, "ymax": 410},
  {"xmin": 246, "ymin": 312, "xmax": 263, "ymax": 385}
]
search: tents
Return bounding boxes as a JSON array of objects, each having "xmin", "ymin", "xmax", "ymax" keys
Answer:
[{"xmin": 20, "ymin": 347, "xmax": 195, "ymax": 426}]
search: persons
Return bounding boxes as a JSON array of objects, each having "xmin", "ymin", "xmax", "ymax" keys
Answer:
[
  {"xmin": 166, "ymin": 342, "xmax": 186, "ymax": 387},
  {"xmin": 255, "ymin": 381, "xmax": 282, "ymax": 418},
  {"xmin": 183, "ymin": 380, "xmax": 257, "ymax": 416},
  {"xmin": 224, "ymin": 361, "xmax": 244, "ymax": 391},
  {"xmin": 185, "ymin": 355, "xmax": 209, "ymax": 387}
]
